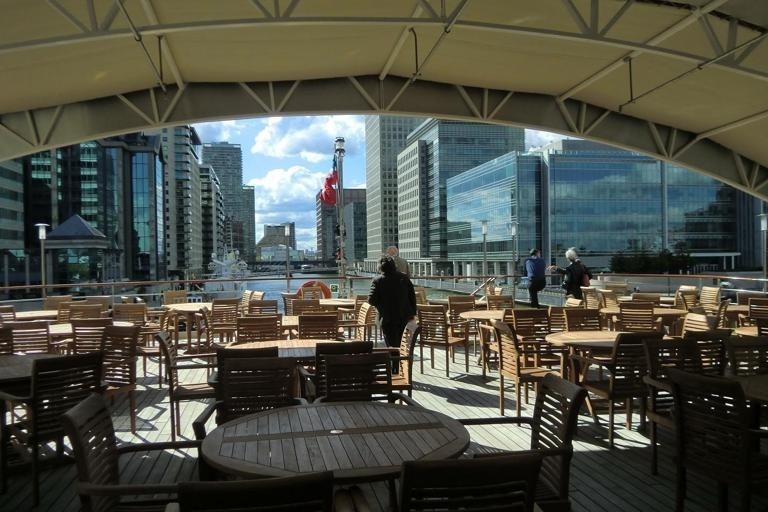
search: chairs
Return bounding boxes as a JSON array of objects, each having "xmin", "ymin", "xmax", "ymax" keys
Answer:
[{"xmin": 0, "ymin": 279, "xmax": 768, "ymax": 510}]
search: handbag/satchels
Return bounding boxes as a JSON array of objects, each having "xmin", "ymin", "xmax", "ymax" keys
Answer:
[
  {"xmin": 581, "ymin": 272, "xmax": 590, "ymax": 286},
  {"xmin": 380, "ymin": 313, "xmax": 406, "ymax": 333},
  {"xmin": 517, "ymin": 277, "xmax": 532, "ymax": 289}
]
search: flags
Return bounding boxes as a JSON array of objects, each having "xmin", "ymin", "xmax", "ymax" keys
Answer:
[{"xmin": 320, "ymin": 157, "xmax": 337, "ymax": 205}]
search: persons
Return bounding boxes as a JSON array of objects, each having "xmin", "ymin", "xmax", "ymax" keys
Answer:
[
  {"xmin": 386, "ymin": 246, "xmax": 410, "ymax": 279},
  {"xmin": 562, "ymin": 251, "xmax": 586, "ymax": 299},
  {"xmin": 524, "ymin": 249, "xmax": 546, "ymax": 308},
  {"xmin": 369, "ymin": 257, "xmax": 416, "ymax": 374},
  {"xmin": 548, "ymin": 247, "xmax": 593, "ymax": 280}
]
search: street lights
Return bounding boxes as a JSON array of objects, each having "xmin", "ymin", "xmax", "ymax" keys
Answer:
[
  {"xmin": 505, "ymin": 218, "xmax": 521, "ymax": 314},
  {"xmin": 326, "ymin": 132, "xmax": 351, "ymax": 303},
  {"xmin": 34, "ymin": 219, "xmax": 54, "ymax": 303},
  {"xmin": 276, "ymin": 217, "xmax": 295, "ymax": 293},
  {"xmin": 259, "ymin": 246, "xmax": 261, "ymax": 263},
  {"xmin": 477, "ymin": 215, "xmax": 493, "ymax": 297},
  {"xmin": 756, "ymin": 212, "xmax": 767, "ymax": 293}
]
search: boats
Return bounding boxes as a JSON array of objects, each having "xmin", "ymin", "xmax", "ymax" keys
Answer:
[
  {"xmin": 196, "ymin": 222, "xmax": 252, "ymax": 306},
  {"xmin": 259, "ymin": 265, "xmax": 293, "ymax": 277},
  {"xmin": 300, "ymin": 264, "xmax": 313, "ymax": 274}
]
